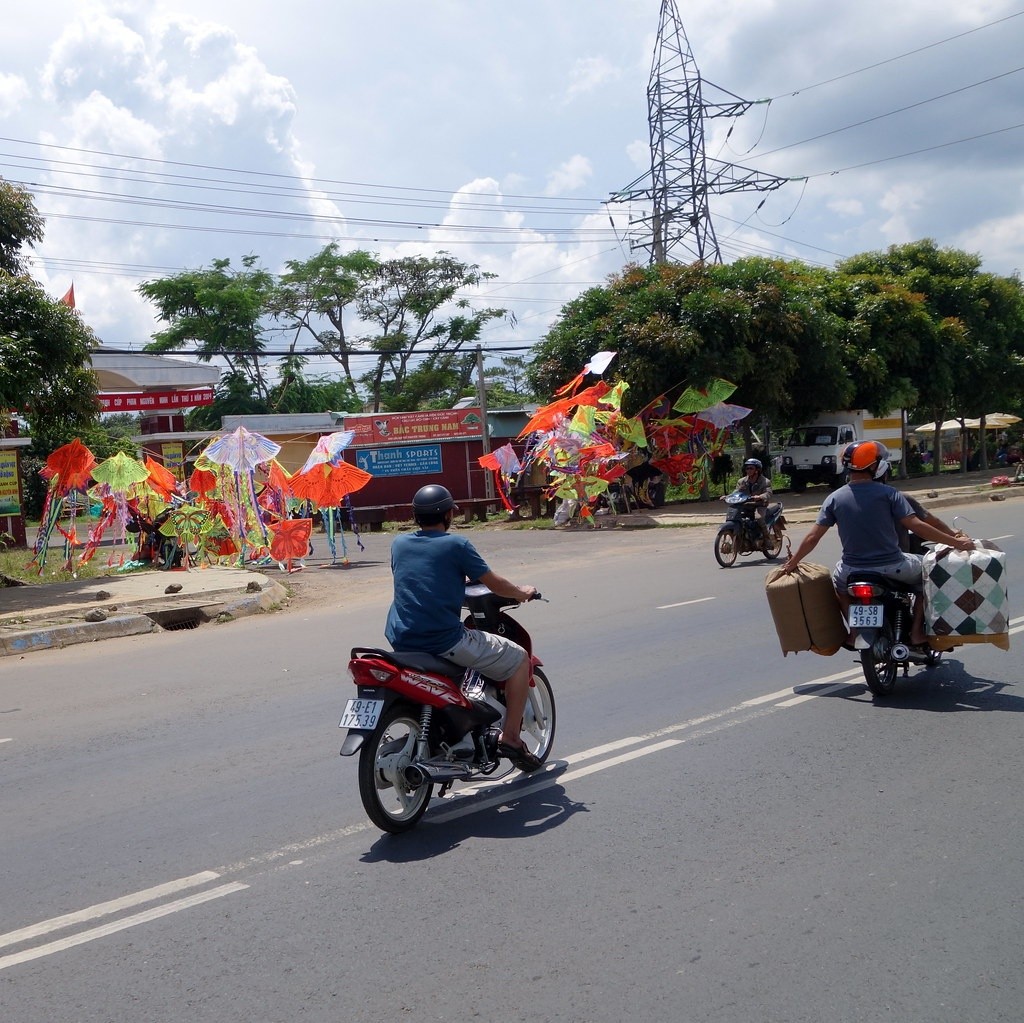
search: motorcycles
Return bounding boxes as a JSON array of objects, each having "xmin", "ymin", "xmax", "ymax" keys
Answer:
[
  {"xmin": 714, "ymin": 490, "xmax": 789, "ymax": 568},
  {"xmin": 339, "ymin": 579, "xmax": 556, "ymax": 835},
  {"xmin": 844, "ymin": 531, "xmax": 943, "ymax": 698}
]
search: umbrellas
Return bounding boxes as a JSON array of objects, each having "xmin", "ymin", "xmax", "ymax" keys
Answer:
[{"xmin": 913, "ymin": 412, "xmax": 1023, "ymax": 452}]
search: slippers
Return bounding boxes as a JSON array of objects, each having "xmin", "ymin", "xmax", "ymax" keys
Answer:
[
  {"xmin": 842, "ymin": 642, "xmax": 855, "ymax": 652},
  {"xmin": 912, "ymin": 638, "xmax": 929, "ymax": 647}
]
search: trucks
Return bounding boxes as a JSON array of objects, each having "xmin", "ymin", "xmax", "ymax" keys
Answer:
[{"xmin": 778, "ymin": 408, "xmax": 908, "ymax": 493}]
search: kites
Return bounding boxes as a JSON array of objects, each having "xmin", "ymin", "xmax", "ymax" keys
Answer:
[
  {"xmin": 477, "ymin": 350, "xmax": 754, "ymax": 531},
  {"xmin": 19, "ymin": 426, "xmax": 374, "ymax": 575}
]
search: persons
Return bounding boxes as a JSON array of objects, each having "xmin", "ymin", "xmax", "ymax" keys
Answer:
[
  {"xmin": 996, "ymin": 436, "xmax": 1023, "ymax": 467},
  {"xmin": 783, "ymin": 439, "xmax": 978, "ymax": 645},
  {"xmin": 720, "ymin": 458, "xmax": 777, "ymax": 551},
  {"xmin": 382, "ymin": 484, "xmax": 543, "ymax": 772}
]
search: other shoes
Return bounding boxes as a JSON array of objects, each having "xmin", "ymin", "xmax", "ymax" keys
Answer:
[
  {"xmin": 763, "ymin": 535, "xmax": 771, "ymax": 547},
  {"xmin": 497, "ymin": 739, "xmax": 541, "ymax": 770}
]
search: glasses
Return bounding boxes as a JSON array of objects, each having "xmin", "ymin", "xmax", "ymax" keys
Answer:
[{"xmin": 746, "ymin": 467, "xmax": 756, "ymax": 470}]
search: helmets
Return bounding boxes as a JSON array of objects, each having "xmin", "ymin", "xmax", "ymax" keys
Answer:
[
  {"xmin": 874, "ymin": 458, "xmax": 892, "ymax": 479},
  {"xmin": 744, "ymin": 458, "xmax": 762, "ymax": 476},
  {"xmin": 412, "ymin": 485, "xmax": 459, "ymax": 517},
  {"xmin": 841, "ymin": 439, "xmax": 889, "ymax": 473}
]
